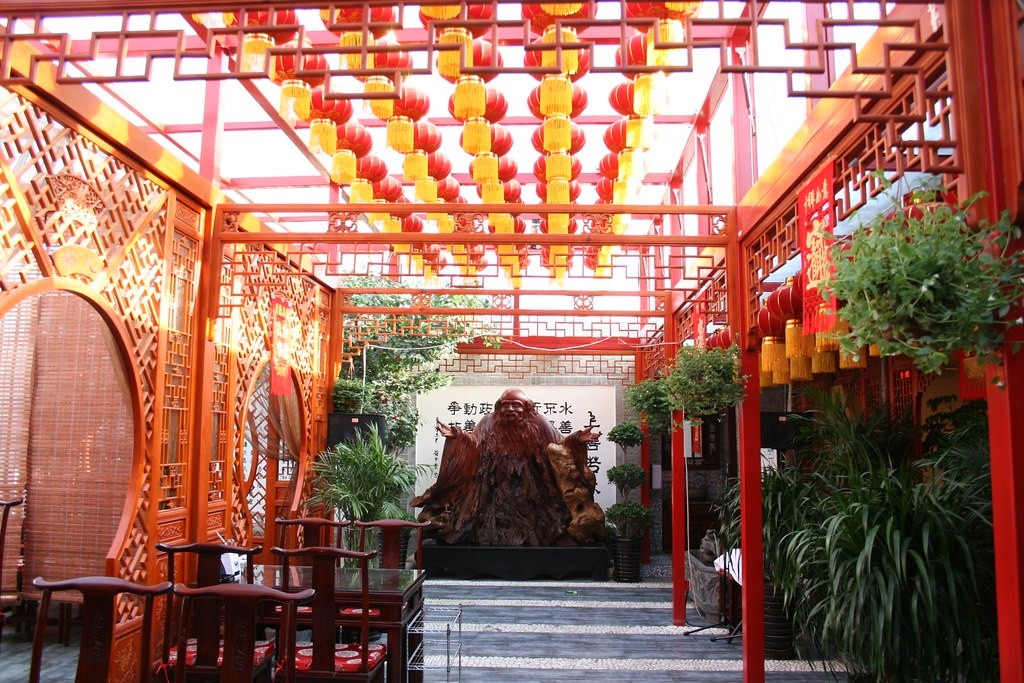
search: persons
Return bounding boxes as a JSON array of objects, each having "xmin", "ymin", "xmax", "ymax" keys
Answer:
[{"xmin": 409, "ymin": 388, "xmax": 606, "ymax": 546}]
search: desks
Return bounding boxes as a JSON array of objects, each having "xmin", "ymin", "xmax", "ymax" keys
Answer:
[{"xmin": 189, "ymin": 565, "xmax": 427, "ymax": 683}]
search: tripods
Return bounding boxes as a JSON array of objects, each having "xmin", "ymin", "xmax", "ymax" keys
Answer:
[{"xmin": 683, "ymin": 541, "xmax": 743, "ymax": 643}]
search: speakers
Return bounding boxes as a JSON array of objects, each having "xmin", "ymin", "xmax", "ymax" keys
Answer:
[
  {"xmin": 326, "ymin": 413, "xmax": 385, "ymax": 447},
  {"xmin": 760, "ymin": 412, "xmax": 813, "ymax": 449}
]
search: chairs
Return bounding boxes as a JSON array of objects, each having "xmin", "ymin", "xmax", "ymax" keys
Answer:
[{"xmin": 0, "ymin": 498, "xmax": 434, "ymax": 683}]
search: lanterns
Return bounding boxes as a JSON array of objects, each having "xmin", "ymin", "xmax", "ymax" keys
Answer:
[
  {"xmin": 223, "ymin": 0, "xmax": 704, "ymax": 287},
  {"xmin": 707, "ymin": 201, "xmax": 945, "ymax": 386}
]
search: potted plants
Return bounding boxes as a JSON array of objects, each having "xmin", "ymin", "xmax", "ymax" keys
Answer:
[
  {"xmin": 710, "ymin": 468, "xmax": 801, "ymax": 659},
  {"xmin": 603, "ymin": 421, "xmax": 655, "ymax": 583},
  {"xmin": 333, "ymin": 378, "xmax": 363, "ymax": 414},
  {"xmin": 377, "ymin": 508, "xmax": 420, "ymax": 569}
]
